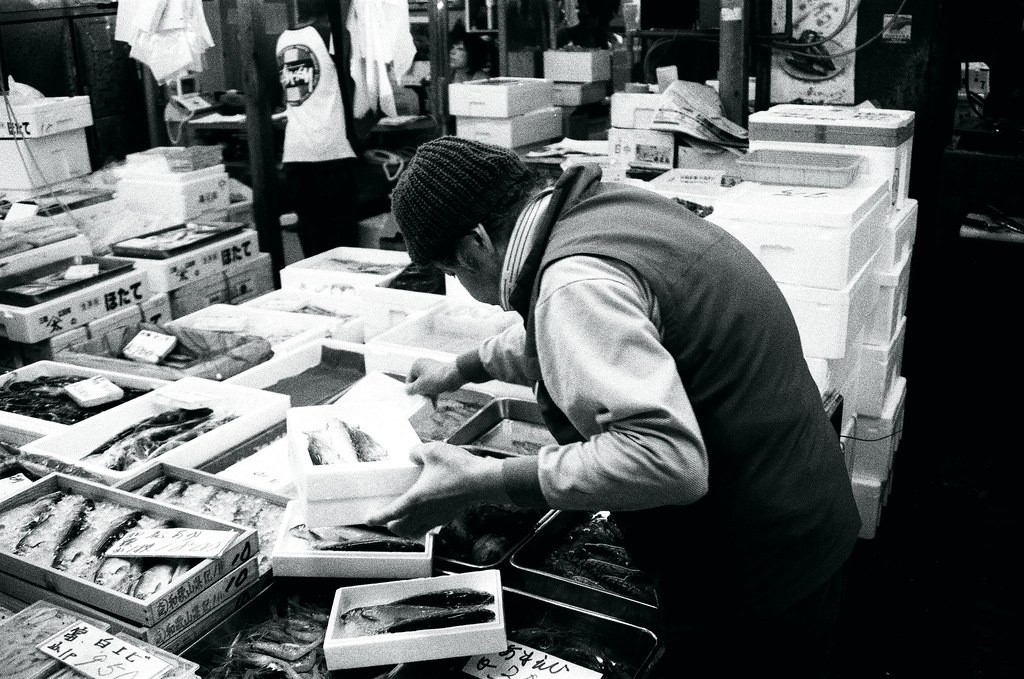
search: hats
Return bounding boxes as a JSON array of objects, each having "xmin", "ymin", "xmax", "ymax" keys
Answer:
[{"xmin": 390, "ymin": 135, "xmax": 528, "ymax": 265}]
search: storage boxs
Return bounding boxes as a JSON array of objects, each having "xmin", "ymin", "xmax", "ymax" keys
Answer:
[{"xmin": 0, "ymin": 44, "xmax": 921, "ymax": 679}]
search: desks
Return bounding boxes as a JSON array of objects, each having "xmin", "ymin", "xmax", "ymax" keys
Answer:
[{"xmin": 169, "ymin": 119, "xmax": 435, "ymax": 150}]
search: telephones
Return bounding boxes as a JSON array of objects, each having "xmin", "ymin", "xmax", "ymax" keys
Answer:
[
  {"xmin": 364, "ymin": 148, "xmax": 413, "ymax": 164},
  {"xmin": 170, "ymin": 92, "xmax": 220, "ymax": 113}
]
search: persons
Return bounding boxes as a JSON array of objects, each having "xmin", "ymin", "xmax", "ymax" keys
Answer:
[
  {"xmin": 364, "ymin": 135, "xmax": 862, "ymax": 679},
  {"xmin": 277, "ymin": 0, "xmax": 373, "ymax": 261},
  {"xmin": 556, "ymin": 0, "xmax": 626, "ymax": 50},
  {"xmin": 448, "ymin": 34, "xmax": 491, "ymax": 82}
]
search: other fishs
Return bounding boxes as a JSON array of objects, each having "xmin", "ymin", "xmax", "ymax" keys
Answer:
[{"xmin": 0, "ymin": 376, "xmax": 643, "ymax": 679}]
